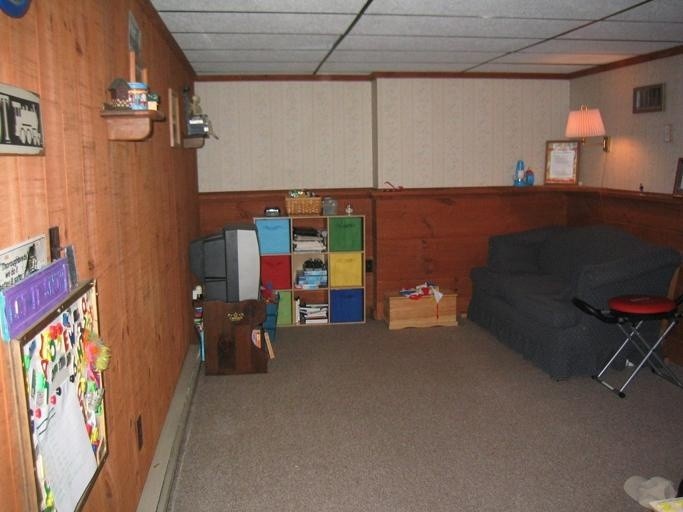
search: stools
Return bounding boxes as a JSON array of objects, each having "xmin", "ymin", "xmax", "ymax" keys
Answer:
[
  {"xmin": 383, "ymin": 289, "xmax": 458, "ymax": 331},
  {"xmin": 572, "ymin": 293, "xmax": 682, "ymax": 398}
]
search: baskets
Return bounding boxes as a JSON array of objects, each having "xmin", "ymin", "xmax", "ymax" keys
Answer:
[{"xmin": 285, "ymin": 196, "xmax": 323, "ymax": 216}]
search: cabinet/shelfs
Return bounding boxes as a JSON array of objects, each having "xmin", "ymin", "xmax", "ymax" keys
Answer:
[{"xmin": 250, "ymin": 214, "xmax": 365, "ymax": 326}]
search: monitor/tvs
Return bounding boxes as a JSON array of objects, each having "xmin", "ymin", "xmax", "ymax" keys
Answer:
[{"xmin": 189, "ymin": 223, "xmax": 261, "ymax": 302}]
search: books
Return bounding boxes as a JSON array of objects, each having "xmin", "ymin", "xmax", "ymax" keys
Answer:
[
  {"xmin": 292, "ymin": 226, "xmax": 328, "ymax": 288},
  {"xmin": 293, "ymin": 296, "xmax": 328, "ymax": 325}
]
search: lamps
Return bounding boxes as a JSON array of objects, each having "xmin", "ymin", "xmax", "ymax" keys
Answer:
[{"xmin": 564, "ymin": 104, "xmax": 609, "ymax": 152}]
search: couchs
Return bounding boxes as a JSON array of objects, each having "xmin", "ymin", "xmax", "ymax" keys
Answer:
[{"xmin": 467, "ymin": 219, "xmax": 679, "ymax": 381}]
